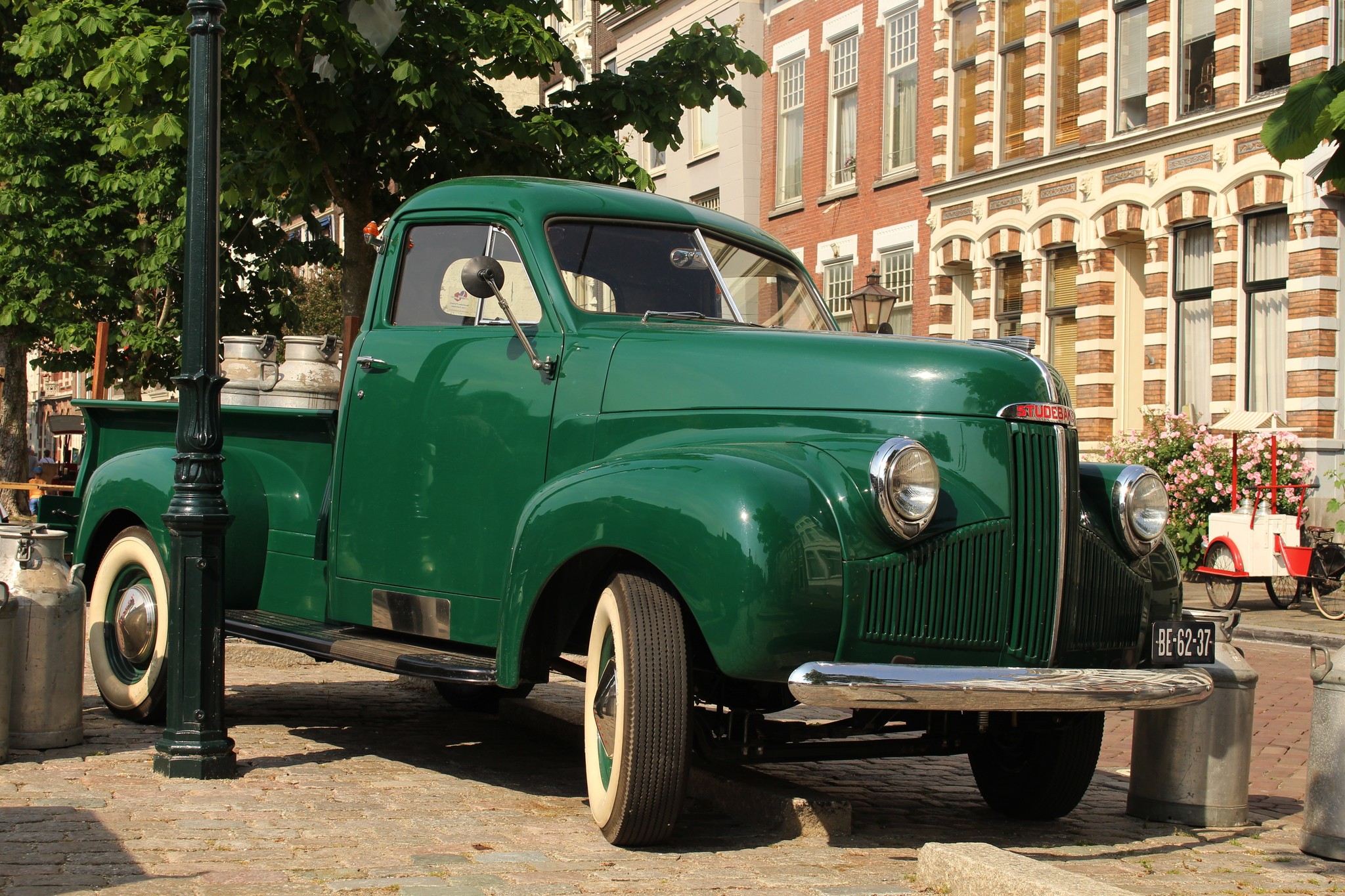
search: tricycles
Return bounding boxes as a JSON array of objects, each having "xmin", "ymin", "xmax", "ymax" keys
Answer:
[{"xmin": 1199, "ymin": 407, "xmax": 1345, "ymax": 625}]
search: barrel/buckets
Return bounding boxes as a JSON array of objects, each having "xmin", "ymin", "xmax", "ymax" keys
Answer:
[
  {"xmin": 257, "ymin": 335, "xmax": 342, "ymax": 409},
  {"xmin": 1, "ymin": 525, "xmax": 87, "ymax": 749},
  {"xmin": 0, "ymin": 577, "xmax": 18, "ymax": 764},
  {"xmin": 220, "ymin": 334, "xmax": 280, "ymax": 408}
]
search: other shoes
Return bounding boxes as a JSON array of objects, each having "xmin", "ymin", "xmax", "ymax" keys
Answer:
[{"xmin": 31, "ymin": 514, "xmax": 38, "ymax": 517}]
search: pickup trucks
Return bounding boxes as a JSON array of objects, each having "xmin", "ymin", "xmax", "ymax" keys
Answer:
[{"xmin": 50, "ymin": 168, "xmax": 1220, "ymax": 852}]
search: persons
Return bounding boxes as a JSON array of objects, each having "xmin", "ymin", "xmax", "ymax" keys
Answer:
[
  {"xmin": 28, "ymin": 466, "xmax": 48, "ymax": 516},
  {"xmin": 27, "ymin": 446, "xmax": 39, "ymax": 480},
  {"xmin": 38, "ymin": 449, "xmax": 56, "ymax": 466},
  {"xmin": 30, "ymin": 449, "xmax": 38, "ymax": 459}
]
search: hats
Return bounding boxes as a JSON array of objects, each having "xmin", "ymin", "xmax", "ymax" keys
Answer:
[
  {"xmin": 28, "ymin": 448, "xmax": 31, "ymax": 452},
  {"xmin": 33, "ymin": 466, "xmax": 43, "ymax": 474}
]
